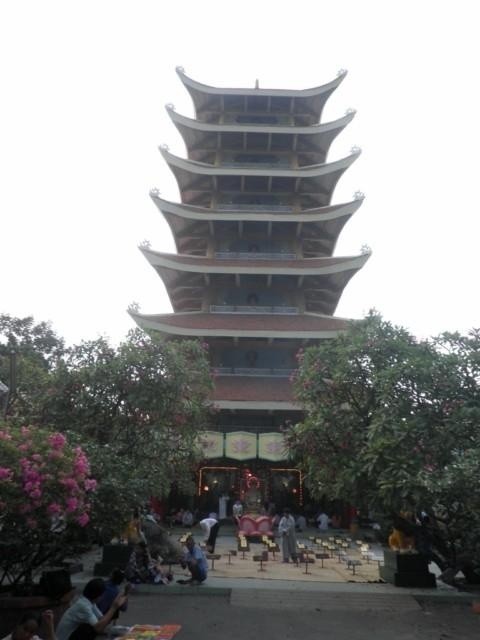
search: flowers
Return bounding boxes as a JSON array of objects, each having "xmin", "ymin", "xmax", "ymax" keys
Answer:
[{"xmin": 1, "ymin": 420, "xmax": 100, "ymax": 590}]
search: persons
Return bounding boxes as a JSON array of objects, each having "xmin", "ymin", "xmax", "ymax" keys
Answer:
[
  {"xmin": 94, "ymin": 568, "xmax": 130, "ymax": 623},
  {"xmin": 53, "ymin": 576, "xmax": 130, "ymax": 640},
  {"xmin": 276, "ymin": 506, "xmax": 300, "ymax": 566},
  {"xmin": 86, "ymin": 492, "xmax": 228, "ymax": 591},
  {"xmin": 0, "ymin": 607, "xmax": 59, "ymax": 640},
  {"xmin": 316, "ymin": 510, "xmax": 332, "ymax": 529}
]
